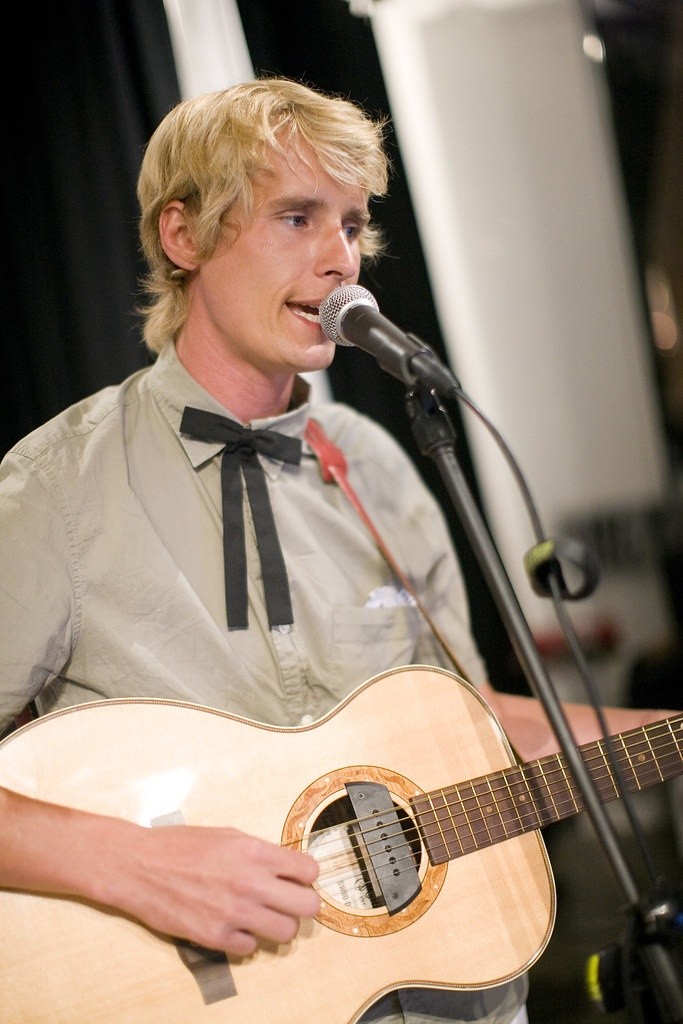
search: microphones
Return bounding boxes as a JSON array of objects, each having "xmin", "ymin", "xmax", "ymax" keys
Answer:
[{"xmin": 321, "ymin": 285, "xmax": 460, "ymax": 397}]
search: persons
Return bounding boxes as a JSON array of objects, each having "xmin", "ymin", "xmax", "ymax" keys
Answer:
[{"xmin": 0, "ymin": 80, "xmax": 683, "ymax": 1023}]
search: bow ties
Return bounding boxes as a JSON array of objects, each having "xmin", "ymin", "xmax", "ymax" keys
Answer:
[{"xmin": 174, "ymin": 405, "xmax": 303, "ymax": 631}]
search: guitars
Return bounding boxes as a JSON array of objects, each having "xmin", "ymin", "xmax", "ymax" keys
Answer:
[{"xmin": 0, "ymin": 662, "xmax": 683, "ymax": 1024}]
